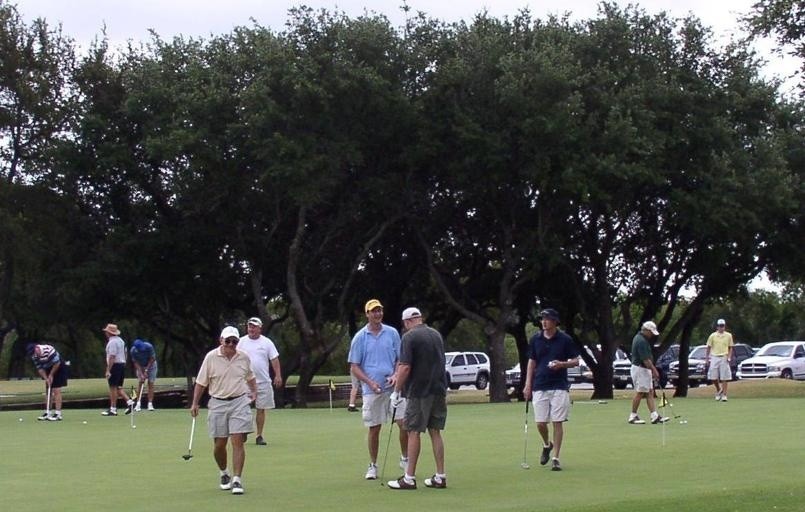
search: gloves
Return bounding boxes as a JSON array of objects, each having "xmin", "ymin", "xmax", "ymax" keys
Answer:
[{"xmin": 389, "ymin": 391, "xmax": 400, "ymax": 406}]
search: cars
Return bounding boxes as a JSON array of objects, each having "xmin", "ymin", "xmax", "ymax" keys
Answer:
[{"xmin": 505, "ymin": 357, "xmax": 522, "ymax": 386}]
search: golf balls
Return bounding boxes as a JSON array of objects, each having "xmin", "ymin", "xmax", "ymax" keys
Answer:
[
  {"xmin": 548, "ymin": 362, "xmax": 556, "ymax": 365},
  {"xmin": 680, "ymin": 420, "xmax": 687, "ymax": 424},
  {"xmin": 83, "ymin": 421, "xmax": 87, "ymax": 424},
  {"xmin": 18, "ymin": 418, "xmax": 23, "ymax": 422}
]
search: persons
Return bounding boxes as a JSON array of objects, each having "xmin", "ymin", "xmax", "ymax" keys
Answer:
[
  {"xmin": 189, "ymin": 325, "xmax": 257, "ymax": 494},
  {"xmin": 523, "ymin": 308, "xmax": 579, "ymax": 470},
  {"xmin": 628, "ymin": 321, "xmax": 669, "ymax": 424},
  {"xmin": 101, "ymin": 323, "xmax": 133, "ymax": 414},
  {"xmin": 27, "ymin": 342, "xmax": 68, "ymax": 420},
  {"xmin": 388, "ymin": 307, "xmax": 447, "ymax": 490},
  {"xmin": 348, "ymin": 299, "xmax": 410, "ymax": 479},
  {"xmin": 236, "ymin": 316, "xmax": 283, "ymax": 445},
  {"xmin": 129, "ymin": 339, "xmax": 158, "ymax": 411},
  {"xmin": 706, "ymin": 318, "xmax": 734, "ymax": 401},
  {"xmin": 348, "ymin": 363, "xmax": 364, "ymax": 412}
]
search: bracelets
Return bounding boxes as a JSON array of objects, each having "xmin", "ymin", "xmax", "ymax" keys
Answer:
[{"xmin": 144, "ymin": 369, "xmax": 148, "ymax": 371}]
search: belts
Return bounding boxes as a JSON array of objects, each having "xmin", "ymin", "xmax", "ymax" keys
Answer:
[{"xmin": 210, "ymin": 393, "xmax": 246, "ymax": 401}]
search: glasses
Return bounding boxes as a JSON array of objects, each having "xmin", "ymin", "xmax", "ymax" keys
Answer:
[{"xmin": 223, "ymin": 338, "xmax": 238, "ymax": 345}]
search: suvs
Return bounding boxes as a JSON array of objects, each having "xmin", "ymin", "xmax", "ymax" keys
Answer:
[
  {"xmin": 566, "ymin": 339, "xmax": 805, "ymax": 389},
  {"xmin": 443, "ymin": 351, "xmax": 491, "ymax": 391}
]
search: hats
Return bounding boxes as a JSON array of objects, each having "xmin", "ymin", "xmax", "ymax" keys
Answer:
[
  {"xmin": 220, "ymin": 325, "xmax": 240, "ymax": 340},
  {"xmin": 536, "ymin": 309, "xmax": 561, "ymax": 327},
  {"xmin": 366, "ymin": 299, "xmax": 421, "ymax": 321},
  {"xmin": 642, "ymin": 320, "xmax": 660, "ymax": 337},
  {"xmin": 246, "ymin": 317, "xmax": 263, "ymax": 327},
  {"xmin": 716, "ymin": 318, "xmax": 726, "ymax": 326},
  {"xmin": 30, "ymin": 325, "xmax": 148, "ymax": 353}
]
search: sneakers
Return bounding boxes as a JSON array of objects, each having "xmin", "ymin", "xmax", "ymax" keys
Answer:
[
  {"xmin": 550, "ymin": 459, "xmax": 561, "ymax": 471},
  {"xmin": 364, "ymin": 458, "xmax": 447, "ymax": 490},
  {"xmin": 242, "ymin": 433, "xmax": 268, "ymax": 446},
  {"xmin": 628, "ymin": 391, "xmax": 730, "ymax": 426},
  {"xmin": 540, "ymin": 441, "xmax": 554, "ymax": 465},
  {"xmin": 38, "ymin": 399, "xmax": 156, "ymax": 420},
  {"xmin": 218, "ymin": 473, "xmax": 243, "ymax": 495}
]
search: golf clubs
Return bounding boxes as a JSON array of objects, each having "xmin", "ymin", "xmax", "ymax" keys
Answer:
[
  {"xmin": 137, "ymin": 379, "xmax": 143, "ymax": 413},
  {"xmin": 130, "ymin": 386, "xmax": 136, "ymax": 429},
  {"xmin": 657, "ymin": 379, "xmax": 681, "ymax": 419},
  {"xmin": 182, "ymin": 417, "xmax": 196, "ymax": 460},
  {"xmin": 381, "ymin": 399, "xmax": 403, "ymax": 486},
  {"xmin": 46, "ymin": 386, "xmax": 57, "ymax": 421},
  {"xmin": 522, "ymin": 399, "xmax": 532, "ymax": 469}
]
